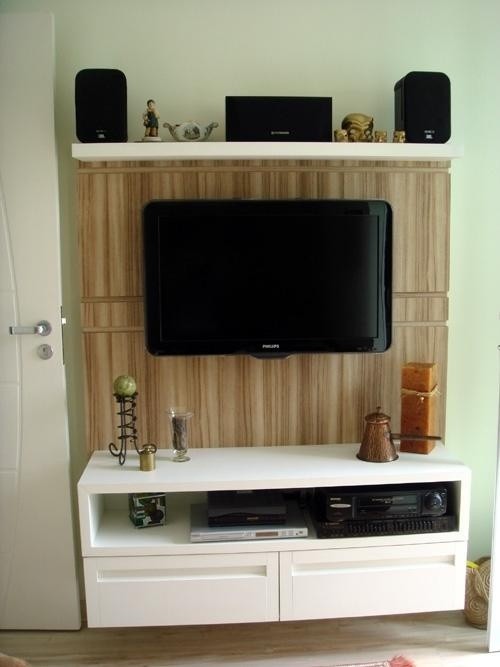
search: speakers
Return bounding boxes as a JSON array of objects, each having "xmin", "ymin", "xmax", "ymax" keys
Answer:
[
  {"xmin": 394, "ymin": 71, "xmax": 451, "ymax": 143},
  {"xmin": 225, "ymin": 96, "xmax": 333, "ymax": 142},
  {"xmin": 75, "ymin": 68, "xmax": 127, "ymax": 143}
]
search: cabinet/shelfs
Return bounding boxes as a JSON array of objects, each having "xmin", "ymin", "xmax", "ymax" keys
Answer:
[{"xmin": 75, "ymin": 439, "xmax": 472, "ymax": 628}]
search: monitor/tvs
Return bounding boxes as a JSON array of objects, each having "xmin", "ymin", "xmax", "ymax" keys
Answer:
[{"xmin": 141, "ymin": 199, "xmax": 393, "ymax": 360}]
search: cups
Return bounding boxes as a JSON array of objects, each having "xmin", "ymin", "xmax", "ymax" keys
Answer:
[{"xmin": 165, "ymin": 407, "xmax": 195, "ymax": 462}]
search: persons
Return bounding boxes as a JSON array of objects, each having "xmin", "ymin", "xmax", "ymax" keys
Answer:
[{"xmin": 141, "ymin": 97, "xmax": 160, "ymax": 136}]
